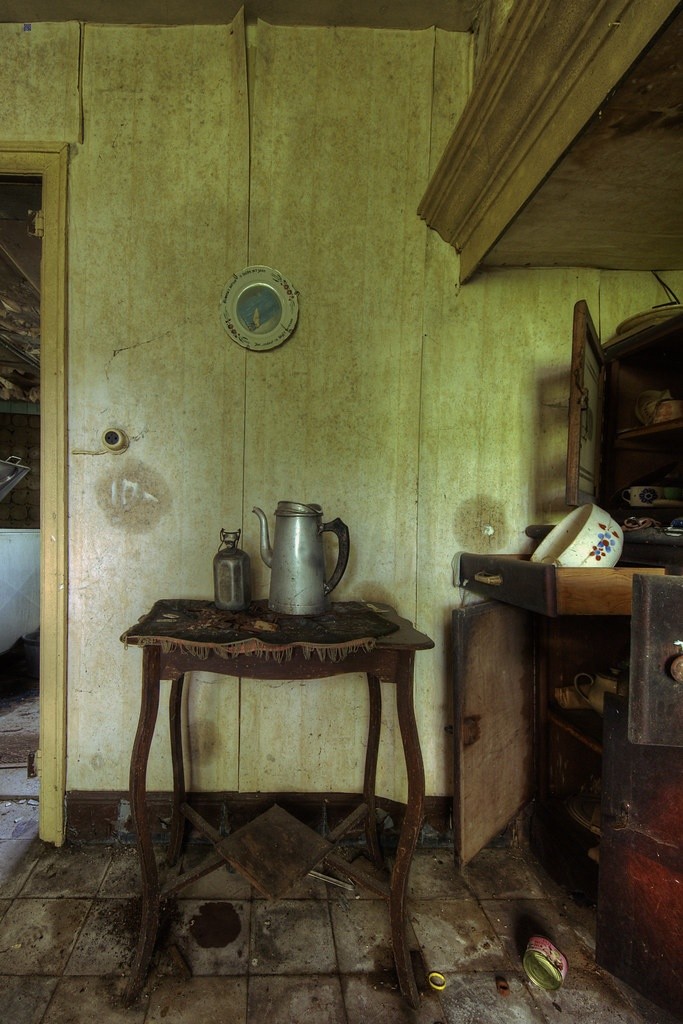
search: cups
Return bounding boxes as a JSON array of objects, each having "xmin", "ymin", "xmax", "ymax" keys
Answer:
[
  {"xmin": 621, "ymin": 486, "xmax": 663, "ymax": 507},
  {"xmin": 662, "ymin": 486, "xmax": 683, "ymax": 499}
]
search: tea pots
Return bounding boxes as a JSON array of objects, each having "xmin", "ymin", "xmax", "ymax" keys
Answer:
[
  {"xmin": 252, "ymin": 500, "xmax": 349, "ymax": 614},
  {"xmin": 574, "ymin": 661, "xmax": 625, "ymax": 719}
]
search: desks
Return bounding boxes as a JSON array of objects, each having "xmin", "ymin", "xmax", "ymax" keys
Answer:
[{"xmin": 119, "ymin": 599, "xmax": 436, "ymax": 1008}]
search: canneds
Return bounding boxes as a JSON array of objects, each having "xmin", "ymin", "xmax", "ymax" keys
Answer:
[{"xmin": 524, "ymin": 934, "xmax": 568, "ymax": 990}]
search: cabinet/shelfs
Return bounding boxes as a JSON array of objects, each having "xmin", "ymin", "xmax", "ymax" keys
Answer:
[{"xmin": 451, "ymin": 299, "xmax": 683, "ymax": 1018}]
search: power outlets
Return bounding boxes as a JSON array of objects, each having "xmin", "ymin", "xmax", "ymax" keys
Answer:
[{"xmin": 103, "ymin": 429, "xmax": 130, "ymax": 455}]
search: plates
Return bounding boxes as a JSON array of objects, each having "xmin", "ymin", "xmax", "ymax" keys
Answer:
[
  {"xmin": 652, "ymin": 498, "xmax": 683, "ymax": 508},
  {"xmin": 570, "ymin": 799, "xmax": 602, "ymax": 839},
  {"xmin": 615, "ymin": 304, "xmax": 683, "ymax": 337}
]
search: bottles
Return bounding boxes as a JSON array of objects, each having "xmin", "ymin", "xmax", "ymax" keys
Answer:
[{"xmin": 213, "ymin": 528, "xmax": 251, "ymax": 611}]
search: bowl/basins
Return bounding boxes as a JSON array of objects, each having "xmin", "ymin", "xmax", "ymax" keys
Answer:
[{"xmin": 530, "ymin": 502, "xmax": 625, "ymax": 570}]
